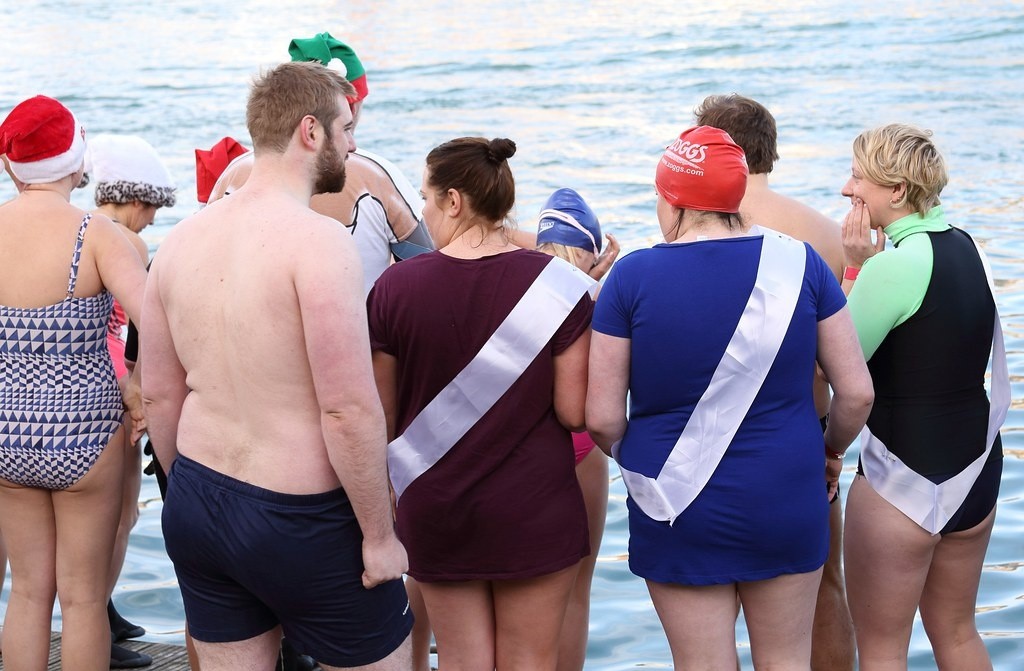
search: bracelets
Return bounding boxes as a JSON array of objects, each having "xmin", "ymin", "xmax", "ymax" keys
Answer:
[
  {"xmin": 828, "ymin": 453, "xmax": 846, "ymax": 459},
  {"xmin": 843, "ymin": 267, "xmax": 860, "ymax": 280}
]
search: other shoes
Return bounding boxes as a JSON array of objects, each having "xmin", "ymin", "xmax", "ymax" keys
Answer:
[
  {"xmin": 107, "ymin": 600, "xmax": 145, "ymax": 642},
  {"xmin": 109, "ymin": 644, "xmax": 153, "ymax": 669}
]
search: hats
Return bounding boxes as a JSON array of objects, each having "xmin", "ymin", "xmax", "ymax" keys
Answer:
[
  {"xmin": 0, "ymin": 94, "xmax": 86, "ymax": 184},
  {"xmin": 536, "ymin": 188, "xmax": 601, "ymax": 255},
  {"xmin": 289, "ymin": 33, "xmax": 368, "ymax": 103},
  {"xmin": 655, "ymin": 126, "xmax": 748, "ymax": 213},
  {"xmin": 195, "ymin": 137, "xmax": 250, "ymax": 203},
  {"xmin": 85, "ymin": 134, "xmax": 177, "ymax": 208}
]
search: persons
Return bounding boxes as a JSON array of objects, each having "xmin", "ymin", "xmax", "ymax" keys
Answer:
[{"xmin": 0, "ymin": 33, "xmax": 1012, "ymax": 671}]
